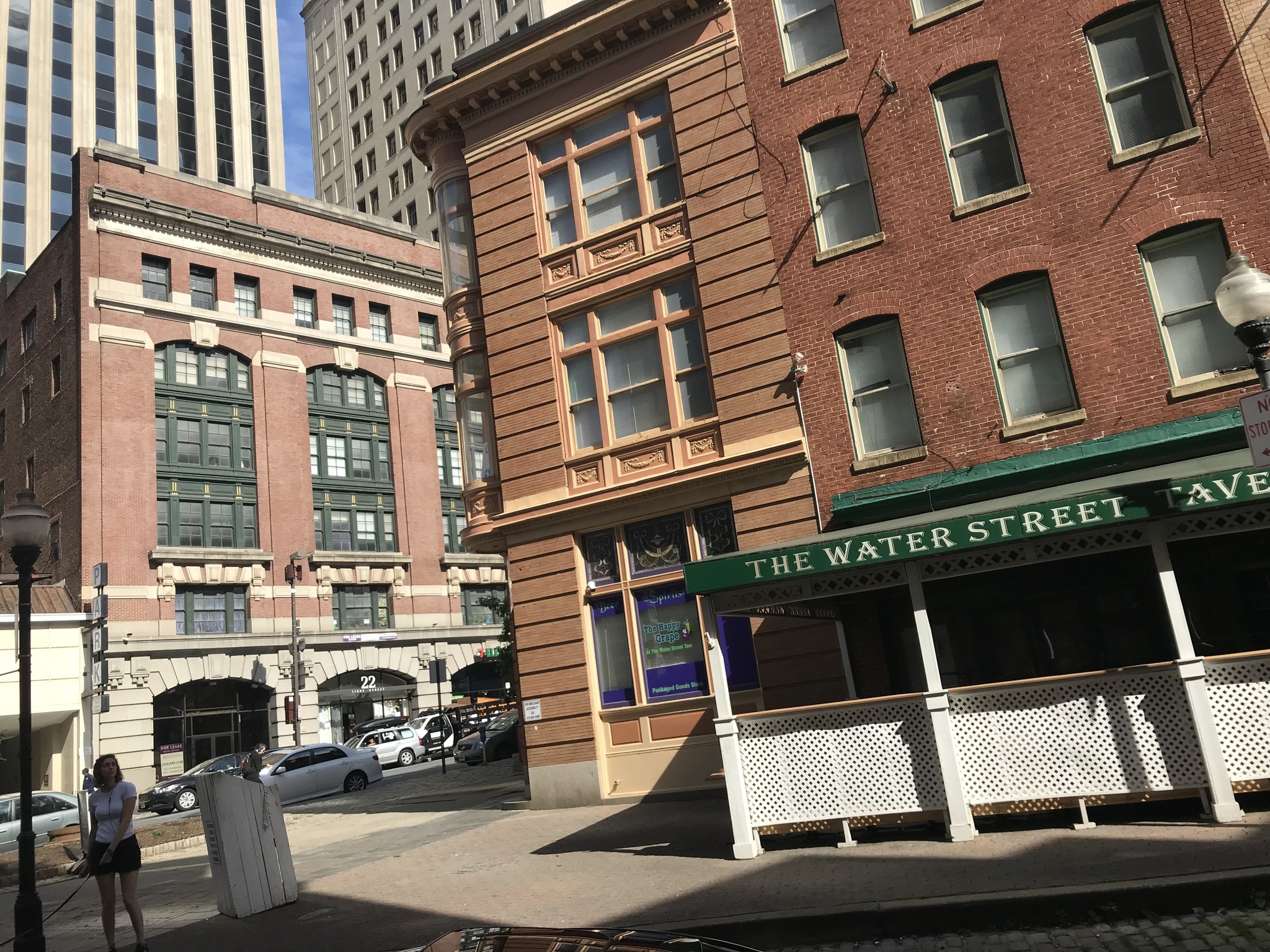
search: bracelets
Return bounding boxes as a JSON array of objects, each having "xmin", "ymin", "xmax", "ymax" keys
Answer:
[{"xmin": 107, "ymin": 848, "xmax": 114, "ymax": 857}]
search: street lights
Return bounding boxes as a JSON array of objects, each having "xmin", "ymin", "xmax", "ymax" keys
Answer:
[
  {"xmin": 285, "ymin": 550, "xmax": 308, "ymax": 747},
  {"xmin": 2, "ymin": 489, "xmax": 54, "ymax": 952},
  {"xmin": 1217, "ymin": 250, "xmax": 1269, "ymax": 393}
]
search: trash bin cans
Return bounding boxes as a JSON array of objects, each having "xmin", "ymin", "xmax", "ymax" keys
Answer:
[{"xmin": 194, "ymin": 770, "xmax": 299, "ymax": 919}]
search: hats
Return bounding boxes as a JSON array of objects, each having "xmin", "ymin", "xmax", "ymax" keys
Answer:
[{"xmin": 81, "ymin": 768, "xmax": 89, "ymax": 772}]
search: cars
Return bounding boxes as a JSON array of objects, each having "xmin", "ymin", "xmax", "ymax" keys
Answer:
[
  {"xmin": 342, "ymin": 715, "xmax": 426, "ymax": 766},
  {"xmin": 138, "ymin": 751, "xmax": 251, "ymax": 815},
  {"xmin": 233, "ymin": 743, "xmax": 385, "ymax": 807},
  {"xmin": 452, "ymin": 708, "xmax": 519, "ymax": 766},
  {"xmin": 0, "ymin": 789, "xmax": 81, "ymax": 853}
]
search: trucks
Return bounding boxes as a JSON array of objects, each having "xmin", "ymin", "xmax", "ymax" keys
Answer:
[{"xmin": 406, "ymin": 710, "xmax": 488, "ymax": 757}]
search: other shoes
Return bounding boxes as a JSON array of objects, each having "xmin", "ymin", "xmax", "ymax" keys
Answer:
[
  {"xmin": 108, "ymin": 947, "xmax": 117, "ymax": 952},
  {"xmin": 136, "ymin": 942, "xmax": 149, "ymax": 952}
]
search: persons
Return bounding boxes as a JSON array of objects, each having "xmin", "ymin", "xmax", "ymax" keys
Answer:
[
  {"xmin": 365, "ymin": 735, "xmax": 377, "ymax": 747},
  {"xmin": 79, "ymin": 753, "xmax": 148, "ymax": 952},
  {"xmin": 81, "ymin": 768, "xmax": 96, "ymax": 794},
  {"xmin": 240, "ymin": 742, "xmax": 267, "ymax": 784}
]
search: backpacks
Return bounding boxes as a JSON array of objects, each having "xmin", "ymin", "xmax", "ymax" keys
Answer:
[{"xmin": 239, "ymin": 752, "xmax": 258, "ymax": 774}]
